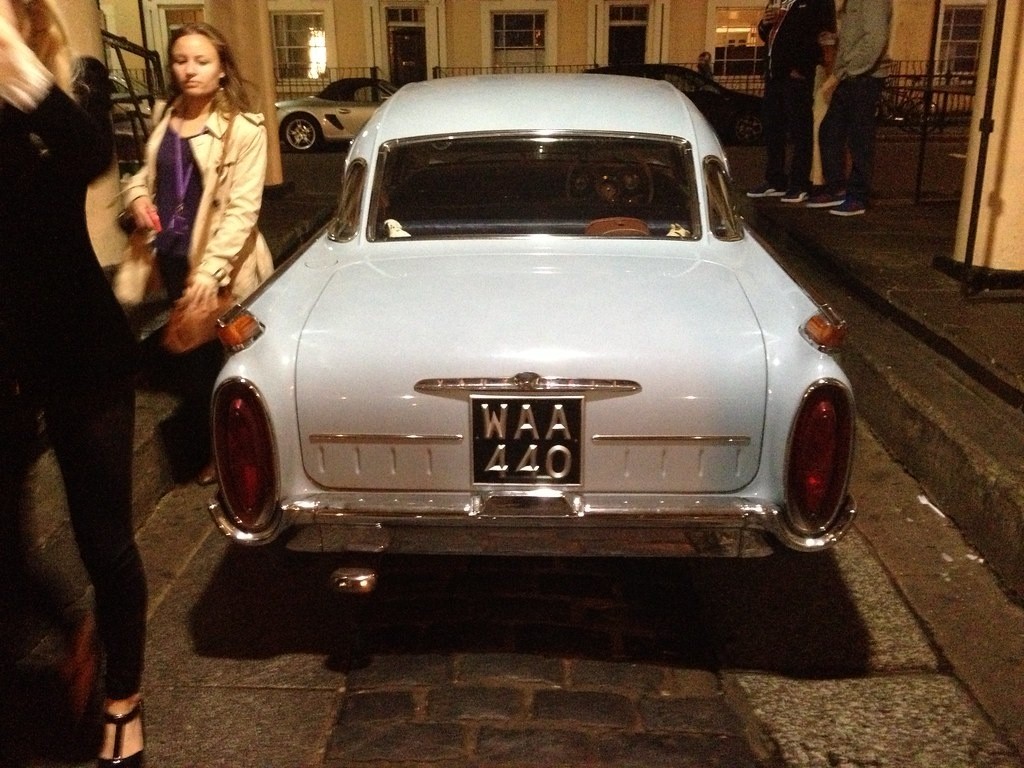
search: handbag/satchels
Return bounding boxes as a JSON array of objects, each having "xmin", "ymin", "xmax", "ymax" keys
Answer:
[{"xmin": 163, "ymin": 288, "xmax": 233, "ymax": 354}]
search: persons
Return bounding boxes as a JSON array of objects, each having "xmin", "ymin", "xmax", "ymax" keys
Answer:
[
  {"xmin": 0, "ymin": 0, "xmax": 150, "ymax": 768},
  {"xmin": 116, "ymin": 22, "xmax": 276, "ymax": 486},
  {"xmin": 697, "ymin": 52, "xmax": 713, "ymax": 80},
  {"xmin": 747, "ymin": 0, "xmax": 893, "ymax": 216}
]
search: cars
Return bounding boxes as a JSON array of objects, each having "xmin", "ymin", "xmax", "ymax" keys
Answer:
[
  {"xmin": 583, "ymin": 63, "xmax": 768, "ymax": 145},
  {"xmin": 109, "ymin": 75, "xmax": 159, "ymax": 137},
  {"xmin": 274, "ymin": 77, "xmax": 398, "ymax": 154},
  {"xmin": 209, "ymin": 72, "xmax": 857, "ymax": 613}
]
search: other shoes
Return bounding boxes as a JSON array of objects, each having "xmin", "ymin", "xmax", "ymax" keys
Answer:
[
  {"xmin": 198, "ymin": 456, "xmax": 220, "ymax": 486},
  {"xmin": 96, "ymin": 697, "xmax": 143, "ymax": 768}
]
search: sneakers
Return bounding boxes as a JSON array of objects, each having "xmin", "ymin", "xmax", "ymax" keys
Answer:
[
  {"xmin": 781, "ymin": 190, "xmax": 809, "ymax": 204},
  {"xmin": 746, "ymin": 184, "xmax": 786, "ymax": 197},
  {"xmin": 828, "ymin": 198, "xmax": 874, "ymax": 217},
  {"xmin": 804, "ymin": 189, "xmax": 847, "ymax": 208}
]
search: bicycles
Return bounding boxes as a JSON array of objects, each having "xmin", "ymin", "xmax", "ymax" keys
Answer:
[{"xmin": 875, "ymin": 74, "xmax": 940, "ymax": 134}]
